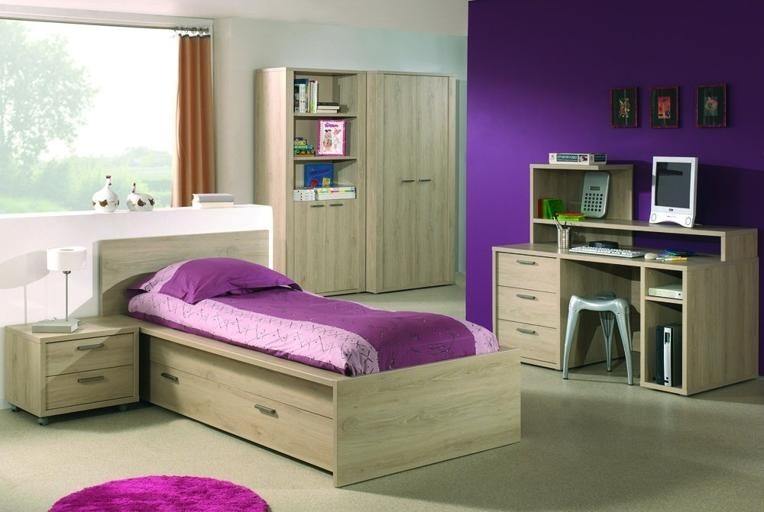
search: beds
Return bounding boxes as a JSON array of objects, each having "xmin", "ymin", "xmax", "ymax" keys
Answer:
[{"xmin": 98, "ymin": 230, "xmax": 521, "ymax": 487}]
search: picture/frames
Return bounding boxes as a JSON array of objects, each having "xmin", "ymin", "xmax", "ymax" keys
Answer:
[
  {"xmin": 608, "ymin": 87, "xmax": 638, "ymax": 128},
  {"xmin": 649, "ymin": 85, "xmax": 679, "ymax": 129},
  {"xmin": 694, "ymin": 83, "xmax": 727, "ymax": 128}
]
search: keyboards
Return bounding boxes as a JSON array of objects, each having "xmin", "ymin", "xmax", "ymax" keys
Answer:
[{"xmin": 569, "ymin": 245, "xmax": 645, "ymax": 258}]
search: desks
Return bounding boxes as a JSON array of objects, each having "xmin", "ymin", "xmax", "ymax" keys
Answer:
[{"xmin": 491, "ymin": 164, "xmax": 760, "ymax": 397}]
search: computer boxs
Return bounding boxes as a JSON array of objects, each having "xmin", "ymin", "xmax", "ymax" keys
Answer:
[{"xmin": 655, "ymin": 324, "xmax": 681, "ymax": 385}]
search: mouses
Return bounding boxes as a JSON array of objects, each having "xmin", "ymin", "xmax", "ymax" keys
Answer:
[{"xmin": 644, "ymin": 252, "xmax": 658, "ymax": 259}]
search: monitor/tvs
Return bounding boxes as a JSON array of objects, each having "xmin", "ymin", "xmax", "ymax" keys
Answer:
[{"xmin": 649, "ymin": 156, "xmax": 699, "ymax": 228}]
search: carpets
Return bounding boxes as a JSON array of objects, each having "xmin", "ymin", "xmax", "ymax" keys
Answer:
[{"xmin": 48, "ymin": 475, "xmax": 272, "ymax": 512}]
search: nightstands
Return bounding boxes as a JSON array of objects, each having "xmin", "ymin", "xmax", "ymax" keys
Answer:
[{"xmin": 5, "ymin": 316, "xmax": 139, "ymax": 425}]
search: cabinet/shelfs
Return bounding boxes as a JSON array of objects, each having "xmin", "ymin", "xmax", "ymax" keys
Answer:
[
  {"xmin": 366, "ymin": 71, "xmax": 457, "ymax": 294},
  {"xmin": 254, "ymin": 67, "xmax": 366, "ymax": 297}
]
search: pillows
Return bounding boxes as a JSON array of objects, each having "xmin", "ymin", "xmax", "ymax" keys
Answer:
[{"xmin": 126, "ymin": 257, "xmax": 303, "ymax": 304}]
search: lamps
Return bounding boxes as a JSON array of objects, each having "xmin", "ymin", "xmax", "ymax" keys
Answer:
[{"xmin": 46, "ymin": 246, "xmax": 86, "ymax": 325}]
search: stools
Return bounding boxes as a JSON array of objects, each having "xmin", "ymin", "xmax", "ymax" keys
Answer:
[{"xmin": 562, "ymin": 291, "xmax": 634, "ymax": 385}]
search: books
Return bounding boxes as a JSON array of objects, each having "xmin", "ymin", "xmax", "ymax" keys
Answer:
[
  {"xmin": 317, "ymin": 119, "xmax": 347, "ymax": 157},
  {"xmin": 191, "ymin": 201, "xmax": 233, "ymax": 209},
  {"xmin": 191, "ymin": 193, "xmax": 233, "ymax": 202},
  {"xmin": 294, "ymin": 77, "xmax": 340, "ymax": 113},
  {"xmin": 31, "ymin": 320, "xmax": 79, "ymax": 333},
  {"xmin": 294, "ymin": 163, "xmax": 355, "ymax": 201}
]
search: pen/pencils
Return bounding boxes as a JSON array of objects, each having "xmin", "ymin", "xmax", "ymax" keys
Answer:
[
  {"xmin": 655, "ymin": 256, "xmax": 688, "ymax": 262},
  {"xmin": 552, "ymin": 217, "xmax": 567, "ymax": 230}
]
